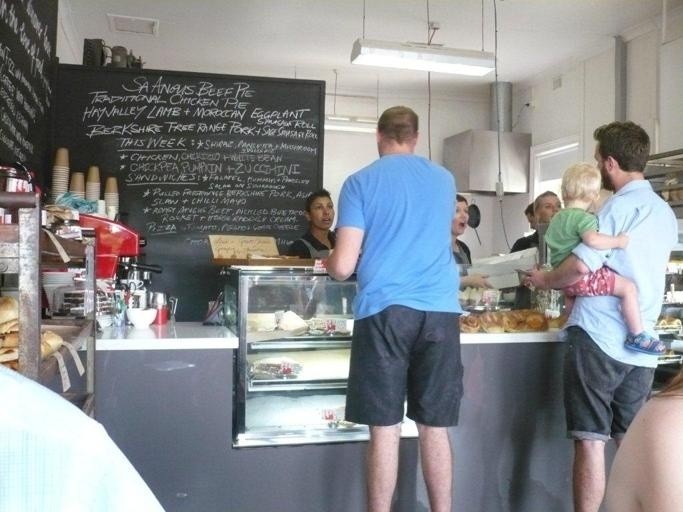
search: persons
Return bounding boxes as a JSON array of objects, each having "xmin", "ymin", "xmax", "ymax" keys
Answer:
[
  {"xmin": 284, "ymin": 188, "xmax": 345, "ymax": 318},
  {"xmin": 523, "ymin": 118, "xmax": 679, "ymax": 511},
  {"xmin": 323, "ymin": 106, "xmax": 463, "ymax": 511},
  {"xmin": 524, "ymin": 204, "xmax": 540, "ymax": 232},
  {"xmin": 509, "ymin": 189, "xmax": 562, "ymax": 307},
  {"xmin": 597, "ymin": 365, "xmax": 682, "ymax": 510},
  {"xmin": 450, "ymin": 193, "xmax": 498, "ymax": 291},
  {"xmin": 543, "ymin": 161, "xmax": 668, "ymax": 355}
]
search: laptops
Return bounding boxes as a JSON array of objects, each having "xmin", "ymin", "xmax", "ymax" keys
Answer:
[
  {"xmin": 0, "ymin": 296, "xmax": 63, "ymax": 370},
  {"xmin": 459, "ymin": 309, "xmax": 549, "ymax": 332}
]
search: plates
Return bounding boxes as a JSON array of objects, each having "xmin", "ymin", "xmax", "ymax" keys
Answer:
[
  {"xmin": 225, "ymin": 267, "xmax": 419, "ymax": 448},
  {"xmin": 643, "ymin": 147, "xmax": 683, "ymax": 396},
  {"xmin": 0, "ymin": 190, "xmax": 95, "ymax": 419}
]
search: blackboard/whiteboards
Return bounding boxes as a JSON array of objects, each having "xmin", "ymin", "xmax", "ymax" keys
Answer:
[
  {"xmin": 1, "ymin": 1, "xmax": 60, "ymax": 199},
  {"xmin": 59, "ymin": 62, "xmax": 325, "ymax": 322}
]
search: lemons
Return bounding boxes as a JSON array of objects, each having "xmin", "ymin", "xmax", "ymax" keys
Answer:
[{"xmin": 148, "ymin": 291, "xmax": 178, "ymax": 326}]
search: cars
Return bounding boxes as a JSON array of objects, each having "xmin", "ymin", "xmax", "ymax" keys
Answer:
[{"xmin": 51, "ymin": 147, "xmax": 119, "ymax": 222}]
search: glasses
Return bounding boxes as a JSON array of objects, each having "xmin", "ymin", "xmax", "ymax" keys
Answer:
[
  {"xmin": 352, "ymin": 0, "xmax": 499, "ymax": 77},
  {"xmin": 324, "ymin": 68, "xmax": 380, "ymax": 133}
]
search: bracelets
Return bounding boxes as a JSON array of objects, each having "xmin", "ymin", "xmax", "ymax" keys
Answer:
[{"xmin": 127, "ymin": 308, "xmax": 157, "ymax": 328}]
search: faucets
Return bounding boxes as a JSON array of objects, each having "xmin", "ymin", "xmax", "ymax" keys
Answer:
[{"xmin": 623, "ymin": 332, "xmax": 669, "ymax": 355}]
